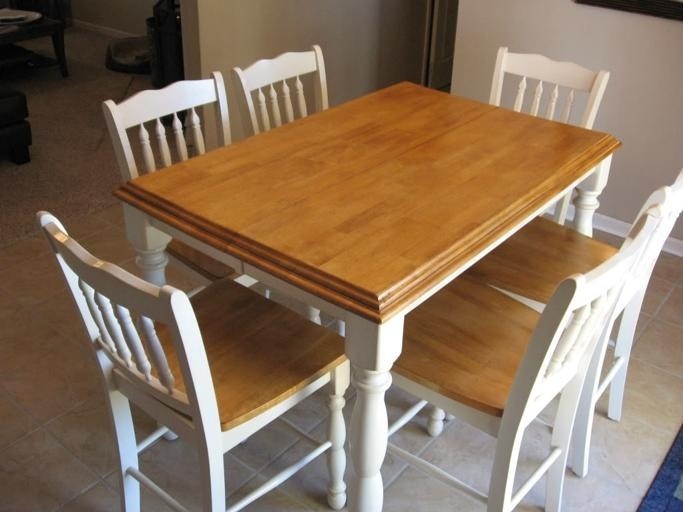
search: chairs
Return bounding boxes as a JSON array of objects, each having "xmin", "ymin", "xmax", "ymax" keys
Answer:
[
  {"xmin": 232, "ymin": 44, "xmax": 329, "ymax": 138},
  {"xmin": 487, "ymin": 45, "xmax": 611, "ymax": 225},
  {"xmin": 102, "ymin": 68, "xmax": 232, "ymax": 281},
  {"xmin": 37, "ymin": 208, "xmax": 350, "ymax": 512},
  {"xmin": 463, "ymin": 165, "xmax": 683, "ymax": 476},
  {"xmin": 388, "ymin": 206, "xmax": 663, "ymax": 511}
]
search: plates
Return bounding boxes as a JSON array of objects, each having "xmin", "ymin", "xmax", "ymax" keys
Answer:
[{"xmin": 0, "ymin": 9, "xmax": 42, "ymax": 25}]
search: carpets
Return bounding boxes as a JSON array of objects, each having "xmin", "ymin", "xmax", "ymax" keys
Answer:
[
  {"xmin": 637, "ymin": 421, "xmax": 682, "ymax": 511},
  {"xmin": 0, "ymin": 25, "xmax": 199, "ymax": 249}
]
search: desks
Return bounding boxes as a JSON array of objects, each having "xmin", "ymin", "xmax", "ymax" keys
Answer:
[{"xmin": 109, "ymin": 81, "xmax": 622, "ymax": 511}]
